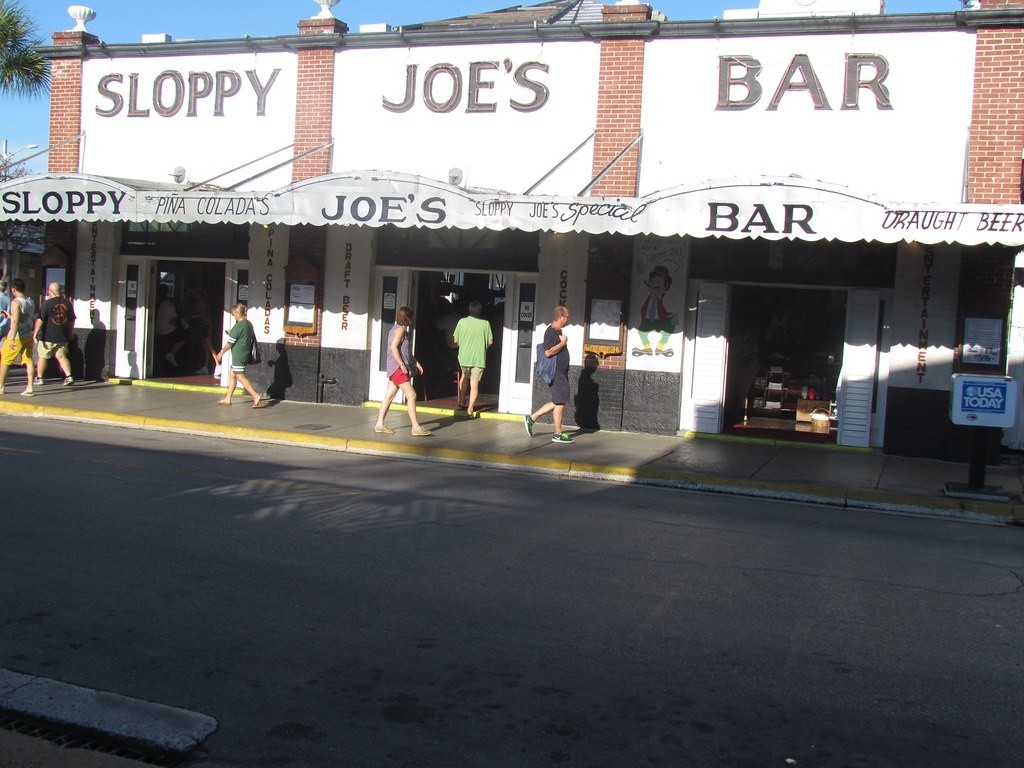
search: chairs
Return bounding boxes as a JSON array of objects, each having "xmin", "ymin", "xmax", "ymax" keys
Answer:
[{"xmin": 828, "ymin": 399, "xmax": 838, "ymax": 434}]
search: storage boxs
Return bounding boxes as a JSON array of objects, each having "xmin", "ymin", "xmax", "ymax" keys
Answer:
[{"xmin": 796, "ymin": 399, "xmax": 829, "ymax": 423}]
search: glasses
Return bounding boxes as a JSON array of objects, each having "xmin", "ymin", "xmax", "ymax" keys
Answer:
[{"xmin": 560, "ymin": 315, "xmax": 569, "ymax": 321}]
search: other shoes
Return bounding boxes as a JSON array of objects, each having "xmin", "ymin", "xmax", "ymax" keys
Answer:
[
  {"xmin": 373, "ymin": 426, "xmax": 394, "ymax": 434},
  {"xmin": 411, "ymin": 427, "xmax": 432, "ymax": 436}
]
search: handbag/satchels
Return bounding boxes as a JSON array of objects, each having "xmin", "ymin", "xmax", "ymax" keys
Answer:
[
  {"xmin": 406, "ymin": 363, "xmax": 419, "ymax": 378},
  {"xmin": 535, "ymin": 336, "xmax": 559, "ymax": 385},
  {"xmin": 248, "ymin": 332, "xmax": 262, "ymax": 365}
]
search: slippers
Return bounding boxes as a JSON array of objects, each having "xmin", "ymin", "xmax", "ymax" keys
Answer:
[
  {"xmin": 20, "ymin": 392, "xmax": 36, "ymax": 396},
  {"xmin": 252, "ymin": 404, "xmax": 267, "ymax": 408},
  {"xmin": 468, "ymin": 415, "xmax": 474, "ymax": 419},
  {"xmin": 217, "ymin": 400, "xmax": 232, "ymax": 406},
  {"xmin": 457, "ymin": 406, "xmax": 465, "ymax": 409},
  {"xmin": 0, "ymin": 391, "xmax": 5, "ymax": 395}
]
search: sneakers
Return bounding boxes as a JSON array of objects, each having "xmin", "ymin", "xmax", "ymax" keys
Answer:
[
  {"xmin": 33, "ymin": 377, "xmax": 44, "ymax": 385},
  {"xmin": 194, "ymin": 366, "xmax": 209, "ymax": 374},
  {"xmin": 523, "ymin": 414, "xmax": 534, "ymax": 438},
  {"xmin": 62, "ymin": 377, "xmax": 74, "ymax": 386},
  {"xmin": 551, "ymin": 433, "xmax": 575, "ymax": 443},
  {"xmin": 214, "ymin": 364, "xmax": 222, "ymax": 376}
]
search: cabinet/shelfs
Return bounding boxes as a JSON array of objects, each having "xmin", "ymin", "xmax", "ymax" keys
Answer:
[{"xmin": 748, "ymin": 365, "xmax": 834, "ymax": 420}]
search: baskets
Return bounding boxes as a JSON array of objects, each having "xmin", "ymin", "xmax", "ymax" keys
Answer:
[{"xmin": 810, "ymin": 408, "xmax": 831, "ymax": 434}]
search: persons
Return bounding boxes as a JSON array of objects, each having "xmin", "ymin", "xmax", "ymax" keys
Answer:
[
  {"xmin": 374, "ymin": 306, "xmax": 431, "ymax": 435},
  {"xmin": 0, "ymin": 278, "xmax": 35, "ymax": 396},
  {"xmin": 156, "ymin": 284, "xmax": 186, "ymax": 366},
  {"xmin": 523, "ymin": 306, "xmax": 574, "ymax": 443},
  {"xmin": 733, "ymin": 320, "xmax": 759, "ymax": 419},
  {"xmin": 217, "ymin": 303, "xmax": 266, "ymax": 408},
  {"xmin": 187, "ymin": 292, "xmax": 217, "ymax": 374},
  {"xmin": 0, "ymin": 280, "xmax": 14, "ymax": 339},
  {"xmin": 32, "ymin": 283, "xmax": 77, "ymax": 386},
  {"xmin": 453, "ymin": 300, "xmax": 493, "ymax": 419},
  {"xmin": 437, "ymin": 302, "xmax": 464, "ymax": 349}
]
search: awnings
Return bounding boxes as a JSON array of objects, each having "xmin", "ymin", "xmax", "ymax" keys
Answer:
[{"xmin": 0, "ymin": 132, "xmax": 1024, "ymax": 246}]
